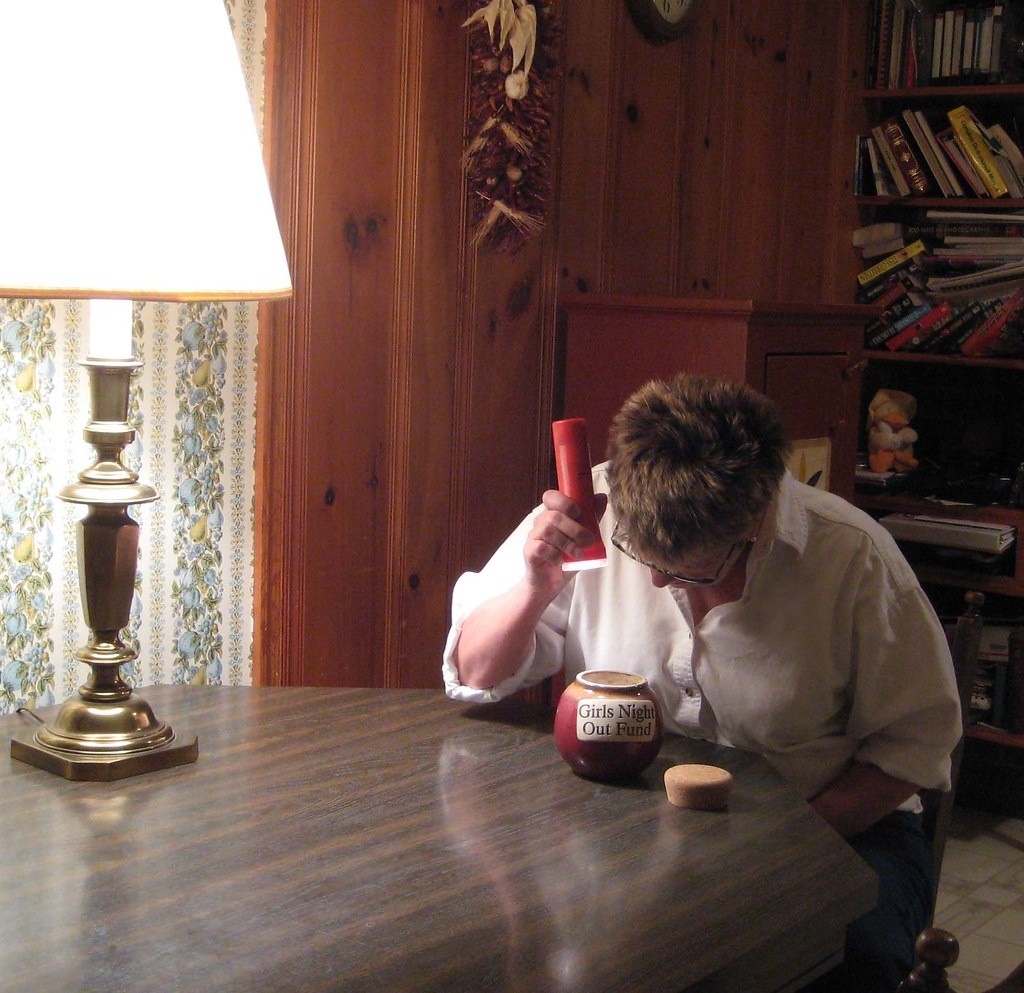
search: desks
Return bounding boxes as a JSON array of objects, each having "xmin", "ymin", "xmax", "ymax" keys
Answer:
[
  {"xmin": 561, "ymin": 291, "xmax": 885, "ymax": 503},
  {"xmin": 0, "ymin": 685, "xmax": 881, "ymax": 993}
]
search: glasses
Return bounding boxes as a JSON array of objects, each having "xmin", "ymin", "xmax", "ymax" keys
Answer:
[{"xmin": 610, "ymin": 514, "xmax": 738, "ymax": 584}]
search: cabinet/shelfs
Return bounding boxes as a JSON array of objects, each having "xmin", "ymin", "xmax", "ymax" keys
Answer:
[{"xmin": 829, "ymin": 0, "xmax": 1024, "ymax": 748}]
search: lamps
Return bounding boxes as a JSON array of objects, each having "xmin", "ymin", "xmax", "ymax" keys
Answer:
[{"xmin": 0, "ymin": 0, "xmax": 293, "ymax": 780}]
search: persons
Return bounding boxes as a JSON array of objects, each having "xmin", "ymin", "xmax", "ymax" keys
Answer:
[{"xmin": 442, "ymin": 372, "xmax": 963, "ymax": 993}]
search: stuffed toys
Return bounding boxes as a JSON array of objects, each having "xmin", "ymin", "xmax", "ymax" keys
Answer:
[{"xmin": 867, "ymin": 389, "xmax": 918, "ymax": 472}]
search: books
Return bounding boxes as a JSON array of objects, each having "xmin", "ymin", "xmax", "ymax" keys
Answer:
[
  {"xmin": 971, "ymin": 628, "xmax": 1024, "ymax": 733},
  {"xmin": 853, "ymin": 105, "xmax": 1024, "ymax": 197},
  {"xmin": 867, "ymin": 0, "xmax": 1024, "ymax": 89},
  {"xmin": 853, "ymin": 211, "xmax": 1024, "ymax": 357}
]
